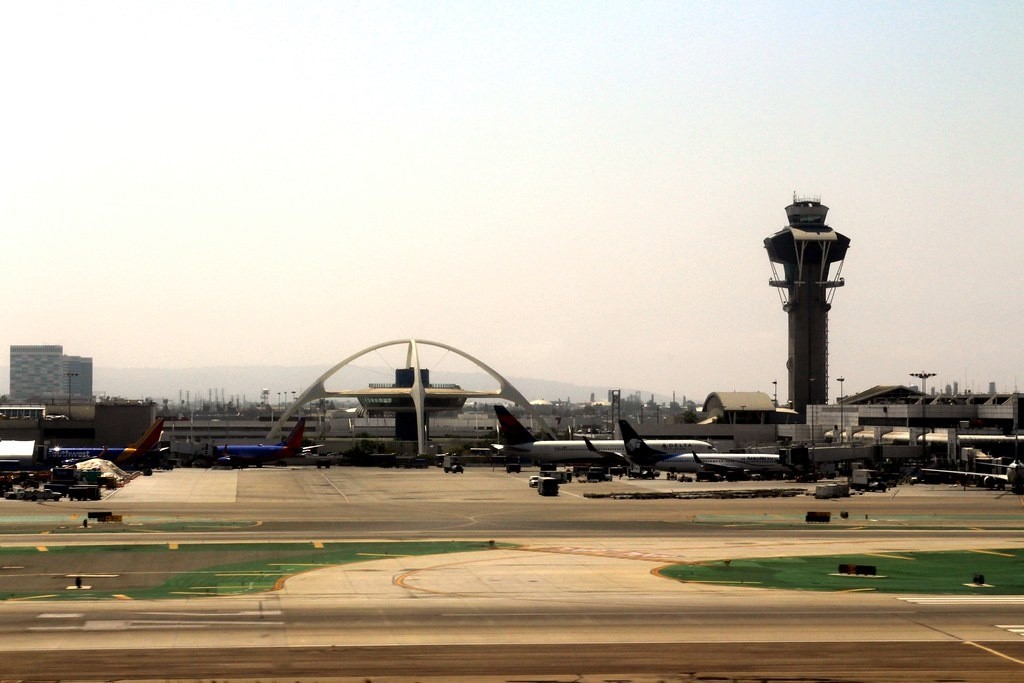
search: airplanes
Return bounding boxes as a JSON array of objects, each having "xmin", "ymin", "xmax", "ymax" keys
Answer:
[
  {"xmin": 469, "ymin": 404, "xmax": 718, "ymax": 482},
  {"xmin": 212, "ymin": 416, "xmax": 326, "ymax": 470},
  {"xmin": 618, "ymin": 418, "xmax": 795, "ymax": 481},
  {"xmin": 46, "ymin": 417, "xmax": 170, "ymax": 476},
  {"xmin": 921, "ymin": 434, "xmax": 1024, "ymax": 495}
]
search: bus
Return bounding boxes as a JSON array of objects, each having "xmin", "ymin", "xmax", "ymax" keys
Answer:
[{"xmin": 538, "ymin": 476, "xmax": 560, "ymax": 496}]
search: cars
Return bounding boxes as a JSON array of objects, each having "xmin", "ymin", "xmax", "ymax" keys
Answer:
[{"xmin": 529, "ymin": 476, "xmax": 540, "ymax": 487}]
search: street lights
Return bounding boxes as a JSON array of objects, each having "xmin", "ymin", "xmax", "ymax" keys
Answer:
[
  {"xmin": 837, "ymin": 378, "xmax": 845, "ymax": 446},
  {"xmin": 66, "ymin": 372, "xmax": 79, "ymax": 418}
]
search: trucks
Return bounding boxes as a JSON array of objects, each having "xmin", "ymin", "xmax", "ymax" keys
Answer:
[
  {"xmin": 27, "ymin": 489, "xmax": 62, "ymax": 501},
  {"xmin": 586, "ymin": 466, "xmax": 605, "ymax": 482},
  {"xmin": 443, "ymin": 455, "xmax": 464, "ymax": 474},
  {"xmin": 852, "ymin": 469, "xmax": 887, "ymax": 493}
]
